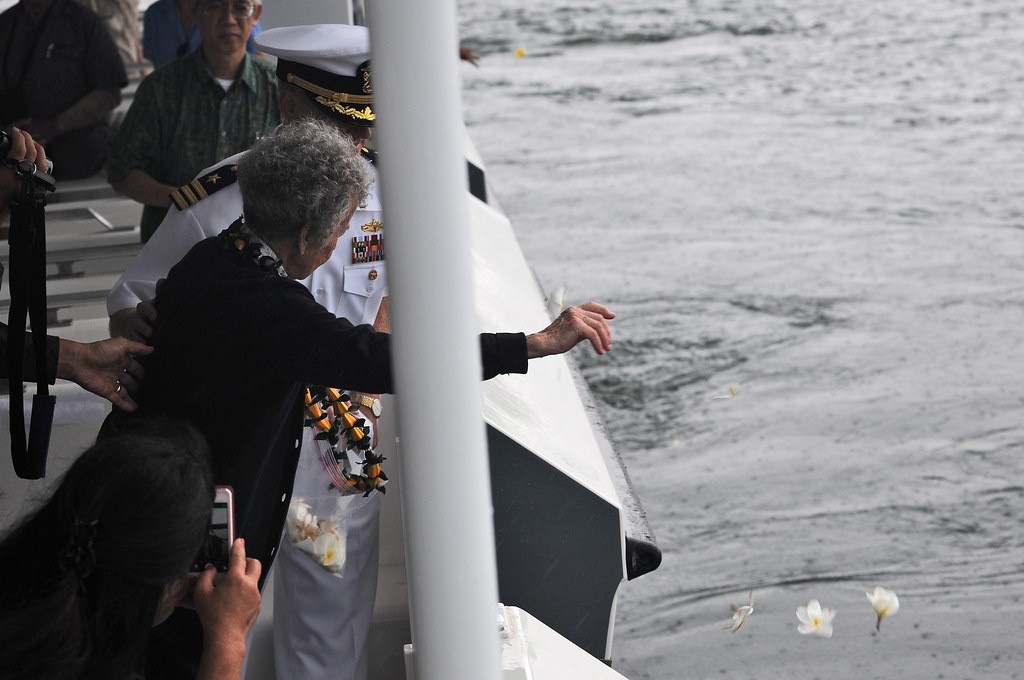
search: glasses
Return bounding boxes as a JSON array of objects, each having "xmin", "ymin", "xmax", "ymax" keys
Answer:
[{"xmin": 193, "ymin": 0, "xmax": 254, "ymax": 19}]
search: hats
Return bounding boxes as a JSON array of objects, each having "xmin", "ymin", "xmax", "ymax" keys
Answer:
[{"xmin": 253, "ymin": 23, "xmax": 376, "ymax": 127}]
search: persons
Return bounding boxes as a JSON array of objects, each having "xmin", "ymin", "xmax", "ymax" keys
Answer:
[
  {"xmin": 0, "ymin": 127, "xmax": 155, "ymax": 414},
  {"xmin": 105, "ymin": 23, "xmax": 389, "ymax": 680},
  {"xmin": 106, "ymin": 0, "xmax": 283, "ymax": 244},
  {"xmin": 82, "ymin": 120, "xmax": 616, "ymax": 587},
  {"xmin": 0, "ymin": 430, "xmax": 263, "ymax": 680},
  {"xmin": 141, "ymin": 0, "xmax": 265, "ymax": 73},
  {"xmin": 0, "ymin": 0, "xmax": 129, "ymax": 182}
]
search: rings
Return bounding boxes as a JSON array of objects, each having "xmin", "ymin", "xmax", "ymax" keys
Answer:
[{"xmin": 116, "ymin": 383, "xmax": 122, "ymax": 392}]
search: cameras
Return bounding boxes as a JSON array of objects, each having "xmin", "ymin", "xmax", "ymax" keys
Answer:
[{"xmin": 0, "ymin": 132, "xmax": 53, "ymax": 178}]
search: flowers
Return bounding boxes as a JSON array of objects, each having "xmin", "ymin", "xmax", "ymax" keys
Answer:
[{"xmin": 303, "ymin": 381, "xmax": 390, "ymax": 501}]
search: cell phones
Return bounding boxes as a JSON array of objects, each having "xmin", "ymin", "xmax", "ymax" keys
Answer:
[{"xmin": 186, "ymin": 485, "xmax": 235, "ymax": 588}]
